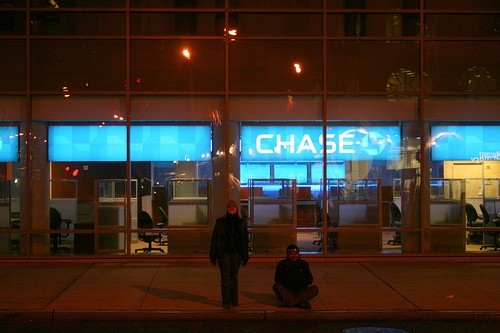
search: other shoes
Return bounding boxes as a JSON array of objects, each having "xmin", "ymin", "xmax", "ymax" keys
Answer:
[
  {"xmin": 224, "ymin": 303, "xmax": 230, "ymax": 309},
  {"xmin": 281, "ymin": 300, "xmax": 288, "ymax": 306},
  {"xmin": 303, "ymin": 302, "xmax": 311, "ymax": 309}
]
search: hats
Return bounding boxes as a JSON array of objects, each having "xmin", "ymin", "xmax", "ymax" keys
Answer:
[{"xmin": 227, "ymin": 200, "xmax": 237, "ymax": 207}]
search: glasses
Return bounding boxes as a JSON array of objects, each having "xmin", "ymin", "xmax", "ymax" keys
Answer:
[{"xmin": 288, "ymin": 249, "xmax": 298, "ymax": 253}]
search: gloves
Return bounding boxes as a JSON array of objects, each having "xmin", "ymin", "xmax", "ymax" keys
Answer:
[
  {"xmin": 210, "ymin": 256, "xmax": 217, "ymax": 266},
  {"xmin": 242, "ymin": 259, "xmax": 249, "ymax": 265}
]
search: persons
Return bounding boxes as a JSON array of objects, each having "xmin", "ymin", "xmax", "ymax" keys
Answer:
[
  {"xmin": 273, "ymin": 244, "xmax": 319, "ymax": 309},
  {"xmin": 210, "ymin": 199, "xmax": 249, "ymax": 309}
]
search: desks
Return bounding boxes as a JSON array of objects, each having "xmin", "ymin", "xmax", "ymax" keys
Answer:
[{"xmin": 298, "ymin": 201, "xmax": 319, "ymax": 227}]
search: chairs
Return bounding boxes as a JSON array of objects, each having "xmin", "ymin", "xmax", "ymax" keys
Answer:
[
  {"xmin": 155, "ymin": 207, "xmax": 169, "ymax": 246},
  {"xmin": 313, "ymin": 204, "xmax": 338, "ymax": 251},
  {"xmin": 136, "ymin": 211, "xmax": 165, "ymax": 253},
  {"xmin": 466, "ymin": 203, "xmax": 500, "ymax": 252},
  {"xmin": 388, "ymin": 202, "xmax": 403, "ymax": 245},
  {"xmin": 50, "ymin": 208, "xmax": 71, "ymax": 252}
]
